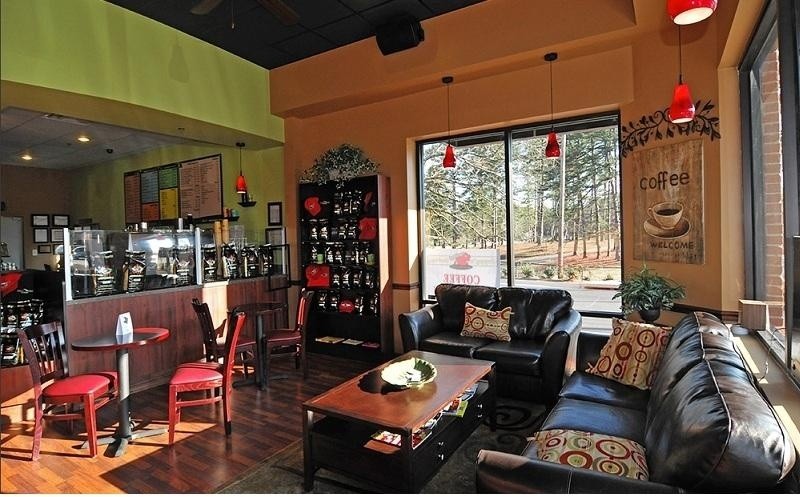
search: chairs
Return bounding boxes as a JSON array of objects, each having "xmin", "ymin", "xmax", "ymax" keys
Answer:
[
  {"xmin": 16, "ymin": 319, "xmax": 121, "ymax": 462},
  {"xmin": 168, "ymin": 293, "xmax": 312, "ymax": 448}
]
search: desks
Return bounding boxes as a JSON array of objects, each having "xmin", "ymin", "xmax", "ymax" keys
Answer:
[{"xmin": 70, "ymin": 326, "xmax": 170, "ymax": 457}]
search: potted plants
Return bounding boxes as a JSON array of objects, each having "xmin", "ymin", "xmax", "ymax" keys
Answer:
[{"xmin": 610, "ymin": 261, "xmax": 687, "ymax": 323}]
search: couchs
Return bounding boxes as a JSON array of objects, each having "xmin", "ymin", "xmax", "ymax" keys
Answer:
[
  {"xmin": 472, "ymin": 308, "xmax": 796, "ymax": 494},
  {"xmin": 398, "ymin": 282, "xmax": 583, "ymax": 414}
]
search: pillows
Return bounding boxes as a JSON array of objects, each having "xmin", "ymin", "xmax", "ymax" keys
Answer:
[
  {"xmin": 460, "ymin": 299, "xmax": 513, "ymax": 343},
  {"xmin": 531, "ymin": 427, "xmax": 652, "ymax": 483},
  {"xmin": 584, "ymin": 316, "xmax": 675, "ymax": 395}
]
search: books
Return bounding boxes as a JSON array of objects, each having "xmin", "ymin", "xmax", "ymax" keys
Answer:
[
  {"xmin": 441, "ymin": 398, "xmax": 462, "ymax": 413},
  {"xmin": 442, "ymin": 400, "xmax": 470, "ymax": 418},
  {"xmin": 371, "ymin": 423, "xmax": 433, "ymax": 450},
  {"xmin": 459, "ymin": 383, "xmax": 479, "ymax": 401},
  {"xmin": 419, "ymin": 412, "xmax": 443, "ymax": 429}
]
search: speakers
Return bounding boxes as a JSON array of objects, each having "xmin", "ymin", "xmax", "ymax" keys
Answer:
[{"xmin": 376, "ymin": 12, "xmax": 425, "ymax": 56}]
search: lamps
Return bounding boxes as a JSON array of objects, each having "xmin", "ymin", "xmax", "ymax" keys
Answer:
[
  {"xmin": 443, "ymin": 76, "xmax": 456, "ymax": 169},
  {"xmin": 666, "ymin": 0, "xmax": 718, "ymax": 126},
  {"xmin": 235, "ymin": 140, "xmax": 248, "ymax": 195},
  {"xmin": 544, "ymin": 51, "xmax": 561, "ymax": 160}
]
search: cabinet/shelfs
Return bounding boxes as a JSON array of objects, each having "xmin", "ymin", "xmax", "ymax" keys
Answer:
[{"xmin": 295, "ymin": 177, "xmax": 395, "ymax": 364}]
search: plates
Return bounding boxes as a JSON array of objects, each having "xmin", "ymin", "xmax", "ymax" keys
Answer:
[{"xmin": 383, "ymin": 358, "xmax": 435, "ymax": 388}]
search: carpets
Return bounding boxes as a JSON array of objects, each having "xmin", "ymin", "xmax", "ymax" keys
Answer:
[{"xmin": 211, "ymin": 396, "xmax": 545, "ymax": 491}]
